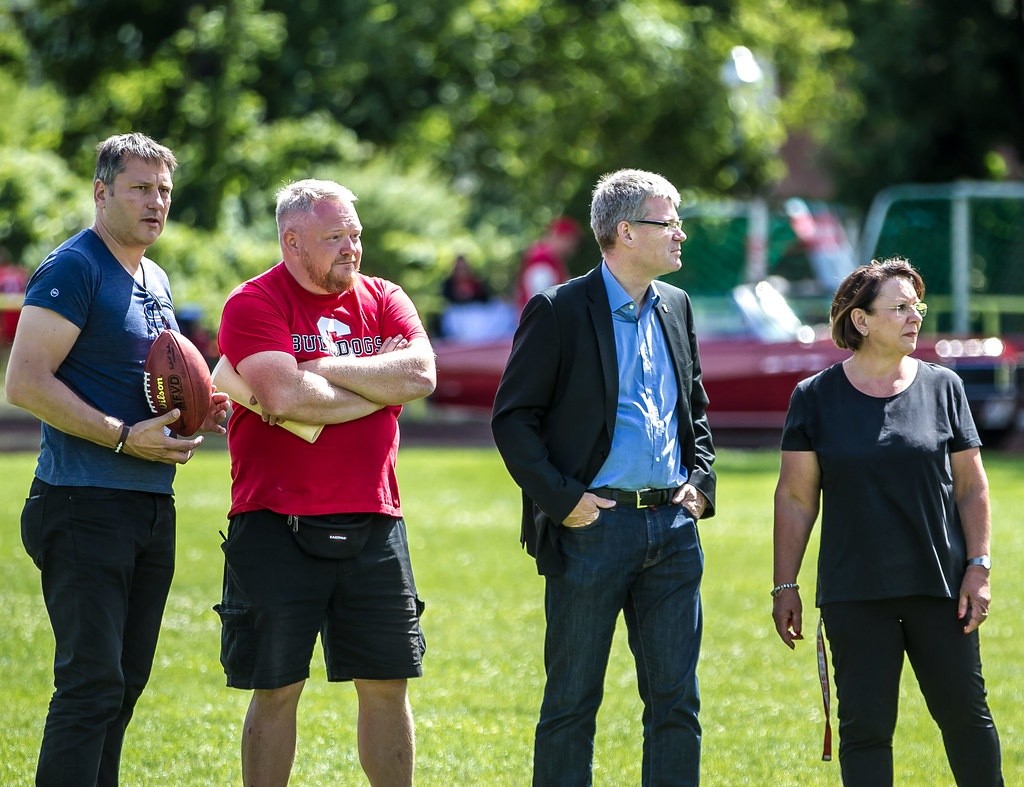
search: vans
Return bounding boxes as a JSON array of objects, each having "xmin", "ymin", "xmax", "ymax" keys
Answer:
[{"xmin": 665, "ymin": 176, "xmax": 1024, "ymax": 342}]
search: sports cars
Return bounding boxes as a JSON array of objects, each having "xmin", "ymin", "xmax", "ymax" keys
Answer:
[{"xmin": 420, "ymin": 277, "xmax": 1015, "ymax": 437}]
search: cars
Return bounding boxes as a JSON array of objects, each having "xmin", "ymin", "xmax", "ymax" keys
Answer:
[{"xmin": 0, "ymin": 258, "xmax": 219, "ymax": 421}]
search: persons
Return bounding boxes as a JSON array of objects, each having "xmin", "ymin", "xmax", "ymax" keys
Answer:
[
  {"xmin": 443, "ymin": 219, "xmax": 581, "ymax": 340},
  {"xmin": 771, "ymin": 257, "xmax": 1006, "ymax": 787},
  {"xmin": 491, "ymin": 168, "xmax": 717, "ymax": 787},
  {"xmin": 4, "ymin": 132, "xmax": 232, "ymax": 787},
  {"xmin": 214, "ymin": 180, "xmax": 437, "ymax": 787}
]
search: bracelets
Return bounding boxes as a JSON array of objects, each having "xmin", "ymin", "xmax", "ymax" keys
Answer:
[
  {"xmin": 771, "ymin": 583, "xmax": 800, "ymax": 595},
  {"xmin": 114, "ymin": 424, "xmax": 130, "ymax": 454}
]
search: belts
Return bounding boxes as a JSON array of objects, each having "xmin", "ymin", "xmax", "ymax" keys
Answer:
[{"xmin": 586, "ymin": 487, "xmax": 679, "ymax": 509}]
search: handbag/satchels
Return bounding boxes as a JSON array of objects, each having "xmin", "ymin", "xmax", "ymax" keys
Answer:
[{"xmin": 277, "ymin": 513, "xmax": 390, "ymax": 560}]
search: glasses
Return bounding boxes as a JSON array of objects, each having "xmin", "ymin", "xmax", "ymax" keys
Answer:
[
  {"xmin": 632, "ymin": 219, "xmax": 683, "ymax": 233},
  {"xmin": 870, "ymin": 303, "xmax": 928, "ymax": 317}
]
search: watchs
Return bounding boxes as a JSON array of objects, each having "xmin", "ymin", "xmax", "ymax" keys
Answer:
[{"xmin": 966, "ymin": 555, "xmax": 992, "ymax": 570}]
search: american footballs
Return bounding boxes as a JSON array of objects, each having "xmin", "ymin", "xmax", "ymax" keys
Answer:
[{"xmin": 142, "ymin": 329, "xmax": 213, "ymax": 438}]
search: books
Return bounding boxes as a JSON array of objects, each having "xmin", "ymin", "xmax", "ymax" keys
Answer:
[{"xmin": 210, "ymin": 354, "xmax": 324, "ymax": 443}]
